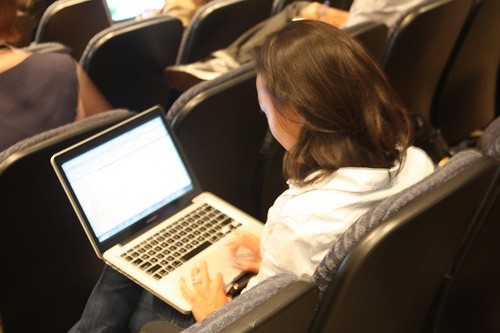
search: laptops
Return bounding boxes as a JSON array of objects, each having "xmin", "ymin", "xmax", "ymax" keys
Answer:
[{"xmin": 49, "ymin": 105, "xmax": 267, "ymax": 315}]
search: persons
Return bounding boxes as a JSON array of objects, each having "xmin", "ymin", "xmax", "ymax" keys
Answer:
[
  {"xmin": 0, "ymin": 1, "xmax": 112, "ymax": 151},
  {"xmin": 166, "ymin": 1, "xmax": 431, "ymax": 85},
  {"xmin": 71, "ymin": 19, "xmax": 436, "ymax": 333}
]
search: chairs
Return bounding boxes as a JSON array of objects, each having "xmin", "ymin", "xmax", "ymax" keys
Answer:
[{"xmin": 0, "ymin": 0, "xmax": 500, "ymax": 333}]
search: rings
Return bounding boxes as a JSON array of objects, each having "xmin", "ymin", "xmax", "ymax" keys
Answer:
[{"xmin": 192, "ymin": 278, "xmax": 200, "ymax": 284}]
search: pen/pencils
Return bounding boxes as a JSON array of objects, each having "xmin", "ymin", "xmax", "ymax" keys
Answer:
[{"xmin": 318, "ymin": 1, "xmax": 331, "ymax": 17}]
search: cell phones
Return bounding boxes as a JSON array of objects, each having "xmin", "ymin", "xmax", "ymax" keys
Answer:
[{"xmin": 228, "ymin": 273, "xmax": 253, "ymax": 297}]
